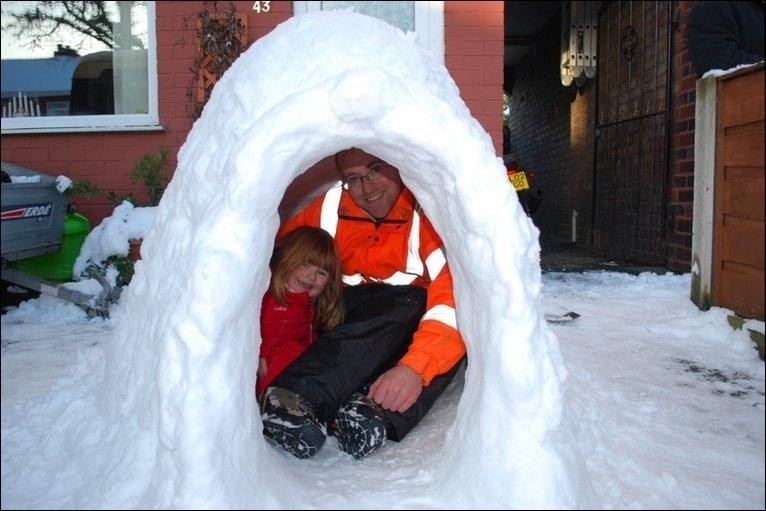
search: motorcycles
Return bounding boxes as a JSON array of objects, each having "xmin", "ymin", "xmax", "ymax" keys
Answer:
[{"xmin": 504, "ymin": 151, "xmax": 544, "ymax": 221}]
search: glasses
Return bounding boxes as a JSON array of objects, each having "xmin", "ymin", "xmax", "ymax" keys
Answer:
[{"xmin": 342, "ymin": 164, "xmax": 390, "ymax": 189}]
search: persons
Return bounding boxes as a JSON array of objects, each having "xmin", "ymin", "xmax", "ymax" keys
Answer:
[
  {"xmin": 259, "ymin": 147, "xmax": 470, "ymax": 465},
  {"xmin": 257, "ymin": 224, "xmax": 348, "ymax": 400}
]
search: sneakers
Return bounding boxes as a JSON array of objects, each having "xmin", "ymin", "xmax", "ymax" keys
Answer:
[
  {"xmin": 335, "ymin": 392, "xmax": 388, "ymax": 461},
  {"xmin": 259, "ymin": 384, "xmax": 325, "ymax": 459}
]
